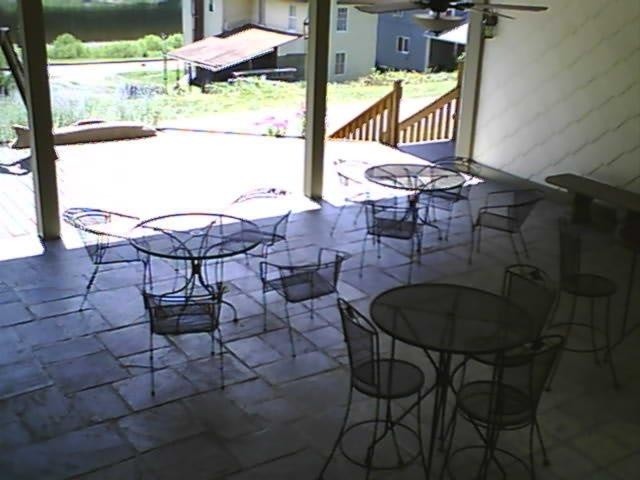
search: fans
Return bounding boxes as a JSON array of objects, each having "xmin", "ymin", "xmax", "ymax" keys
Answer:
[{"xmin": 335, "ymin": 0, "xmax": 548, "ymax": 23}]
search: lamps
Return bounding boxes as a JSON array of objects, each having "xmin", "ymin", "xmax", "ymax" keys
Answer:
[
  {"xmin": 482, "ymin": 15, "xmax": 498, "ymax": 38},
  {"xmin": 412, "ymin": 0, "xmax": 467, "ymax": 37}
]
